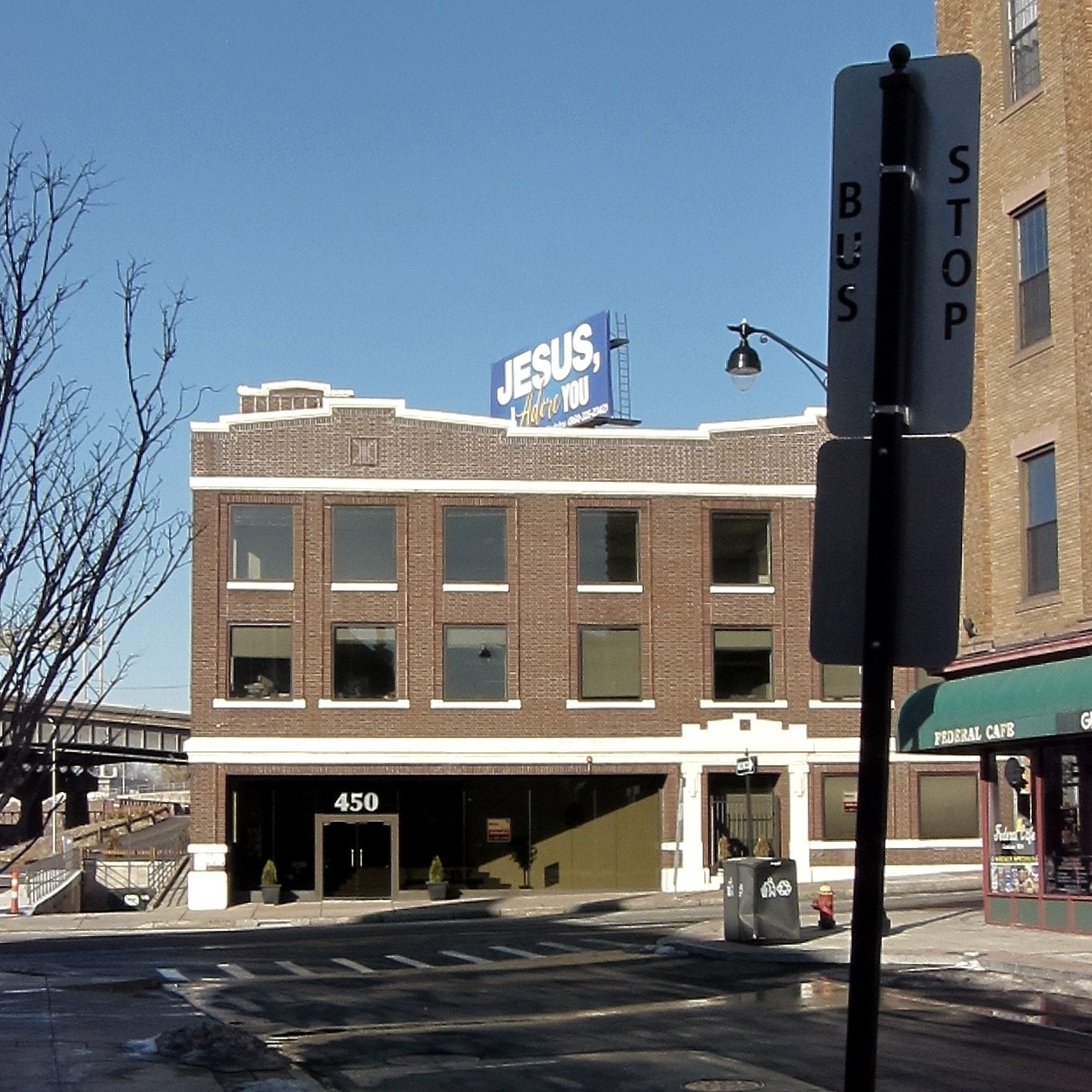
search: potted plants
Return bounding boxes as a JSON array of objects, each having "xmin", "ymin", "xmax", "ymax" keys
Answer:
[
  {"xmin": 426, "ymin": 856, "xmax": 449, "ymax": 900},
  {"xmin": 258, "ymin": 859, "xmax": 283, "ymax": 905}
]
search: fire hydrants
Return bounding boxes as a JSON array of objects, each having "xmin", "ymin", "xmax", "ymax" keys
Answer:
[{"xmin": 811, "ymin": 885, "xmax": 837, "ymax": 929}]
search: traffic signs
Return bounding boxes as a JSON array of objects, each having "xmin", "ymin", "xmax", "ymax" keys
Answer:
[{"xmin": 737, "ymin": 756, "xmax": 755, "ymax": 775}]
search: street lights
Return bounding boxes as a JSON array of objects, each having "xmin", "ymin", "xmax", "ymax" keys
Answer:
[{"xmin": 725, "ymin": 318, "xmax": 892, "ymax": 937}]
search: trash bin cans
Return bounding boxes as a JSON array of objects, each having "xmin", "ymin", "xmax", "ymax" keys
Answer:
[
  {"xmin": 722, "ymin": 856, "xmax": 763, "ymax": 943},
  {"xmin": 737, "ymin": 856, "xmax": 801, "ymax": 945}
]
